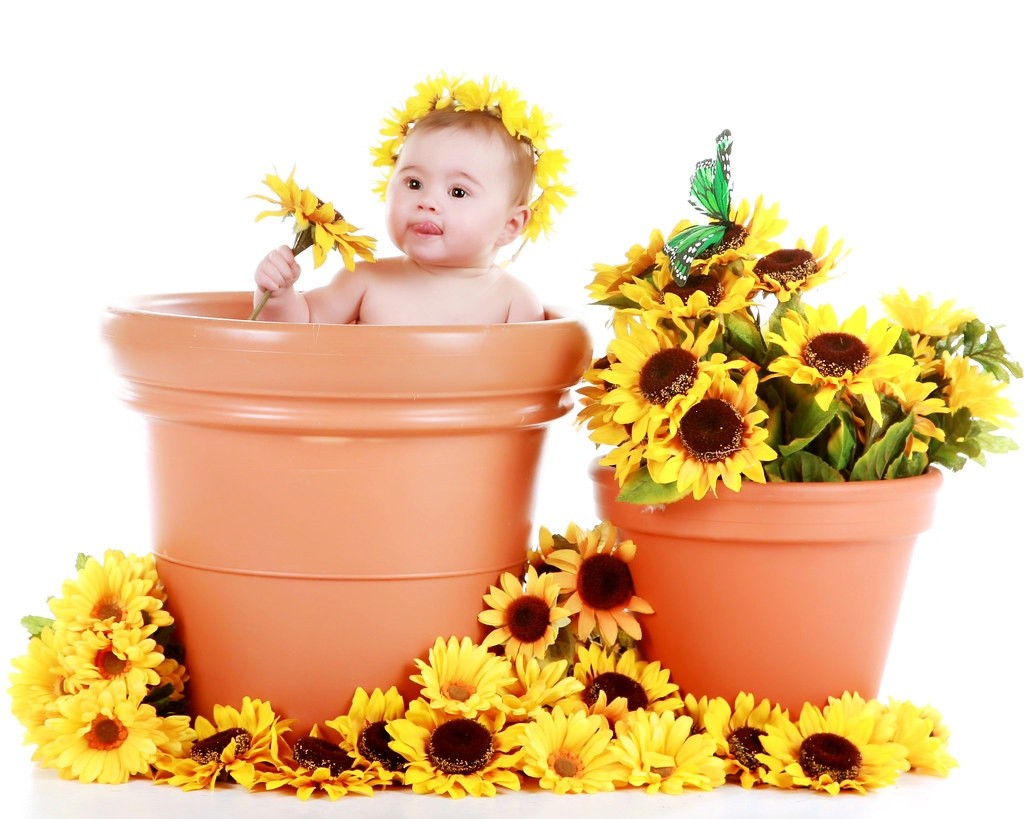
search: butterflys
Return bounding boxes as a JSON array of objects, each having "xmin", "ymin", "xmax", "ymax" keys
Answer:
[{"xmin": 661, "ymin": 128, "xmax": 740, "ymax": 288}]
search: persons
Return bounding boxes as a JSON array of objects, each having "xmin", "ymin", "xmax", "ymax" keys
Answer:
[{"xmin": 250, "ymin": 103, "xmax": 549, "ymax": 326}]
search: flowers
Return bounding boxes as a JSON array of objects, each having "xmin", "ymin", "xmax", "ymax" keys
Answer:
[
  {"xmin": 247, "ymin": 163, "xmax": 378, "ymax": 320},
  {"xmin": 368, "ymin": 69, "xmax": 578, "ymax": 265},
  {"xmin": 574, "ymin": 125, "xmax": 1024, "ymax": 507}
]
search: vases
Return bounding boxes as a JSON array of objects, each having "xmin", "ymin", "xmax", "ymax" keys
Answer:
[
  {"xmin": 588, "ymin": 452, "xmax": 946, "ymax": 718},
  {"xmin": 102, "ymin": 286, "xmax": 594, "ymax": 743}
]
